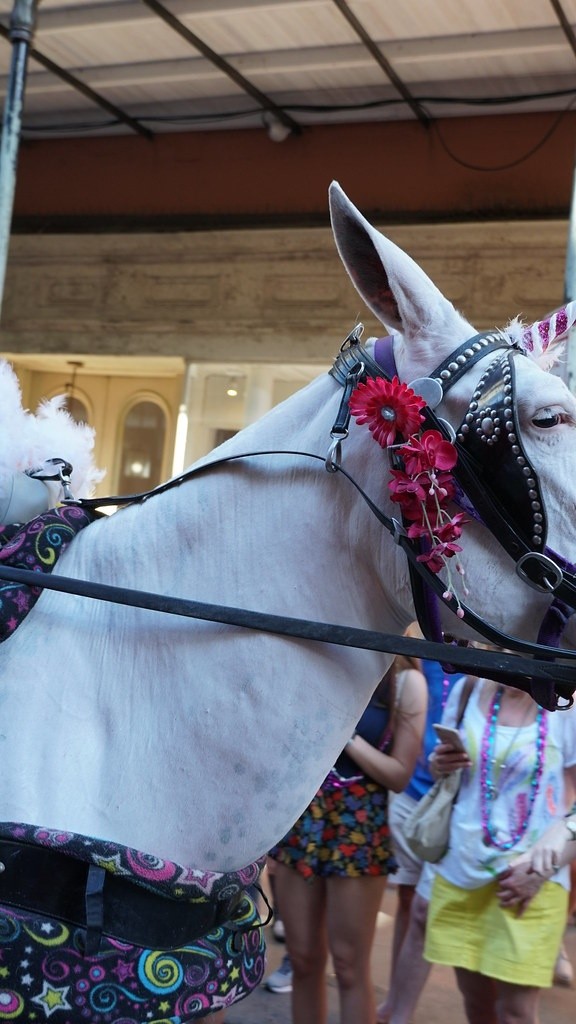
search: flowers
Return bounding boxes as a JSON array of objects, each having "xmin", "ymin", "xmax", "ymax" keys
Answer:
[{"xmin": 349, "ymin": 374, "xmax": 472, "ymax": 621}]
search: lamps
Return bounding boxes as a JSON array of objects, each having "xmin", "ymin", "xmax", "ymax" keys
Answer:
[{"xmin": 124, "ymin": 402, "xmax": 153, "ymax": 483}]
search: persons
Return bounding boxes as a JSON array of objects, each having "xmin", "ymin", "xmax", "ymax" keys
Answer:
[{"xmin": 255, "ymin": 625, "xmax": 575, "ymax": 1024}]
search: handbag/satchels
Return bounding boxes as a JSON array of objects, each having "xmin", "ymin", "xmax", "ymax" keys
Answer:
[{"xmin": 403, "ymin": 679, "xmax": 478, "ymax": 859}]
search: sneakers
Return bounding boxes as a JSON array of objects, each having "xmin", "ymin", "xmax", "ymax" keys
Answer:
[{"xmin": 268, "ymin": 954, "xmax": 295, "ymax": 993}]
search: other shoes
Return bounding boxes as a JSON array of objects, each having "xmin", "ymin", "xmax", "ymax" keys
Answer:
[
  {"xmin": 556, "ymin": 958, "xmax": 573, "ymax": 985},
  {"xmin": 274, "ymin": 908, "xmax": 287, "ymax": 940}
]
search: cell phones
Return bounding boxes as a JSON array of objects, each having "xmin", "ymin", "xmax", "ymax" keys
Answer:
[{"xmin": 433, "ymin": 722, "xmax": 470, "ymax": 762}]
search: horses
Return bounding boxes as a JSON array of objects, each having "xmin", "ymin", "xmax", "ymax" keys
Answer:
[{"xmin": 0, "ymin": 178, "xmax": 575, "ymax": 1024}]
